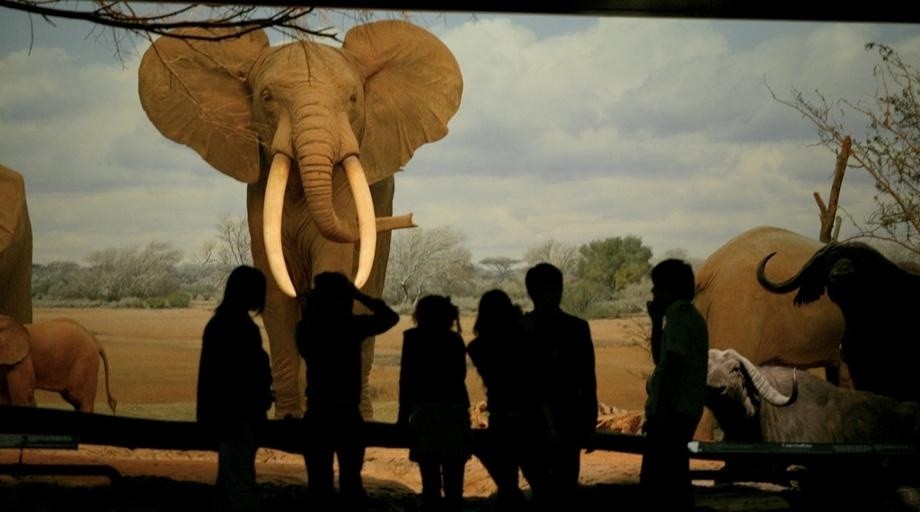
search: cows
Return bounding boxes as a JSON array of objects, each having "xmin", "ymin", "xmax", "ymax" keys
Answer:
[
  {"xmin": 756, "ymin": 240, "xmax": 920, "ymax": 401},
  {"xmin": 644, "ymin": 347, "xmax": 920, "ymax": 512}
]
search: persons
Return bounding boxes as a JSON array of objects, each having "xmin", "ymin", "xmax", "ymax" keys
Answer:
[
  {"xmin": 293, "ymin": 269, "xmax": 400, "ymax": 493},
  {"xmin": 525, "ymin": 262, "xmax": 600, "ymax": 497},
  {"xmin": 195, "ymin": 263, "xmax": 277, "ymax": 483},
  {"xmin": 467, "ymin": 287, "xmax": 524, "ymax": 489},
  {"xmin": 395, "ymin": 292, "xmax": 473, "ymax": 497},
  {"xmin": 637, "ymin": 257, "xmax": 710, "ymax": 485}
]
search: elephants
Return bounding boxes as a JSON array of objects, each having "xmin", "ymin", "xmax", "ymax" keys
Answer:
[
  {"xmin": 137, "ymin": 20, "xmax": 463, "ymax": 421},
  {"xmin": 0, "ymin": 315, "xmax": 117, "ymax": 415},
  {"xmin": 688, "ymin": 227, "xmax": 854, "ymax": 441}
]
